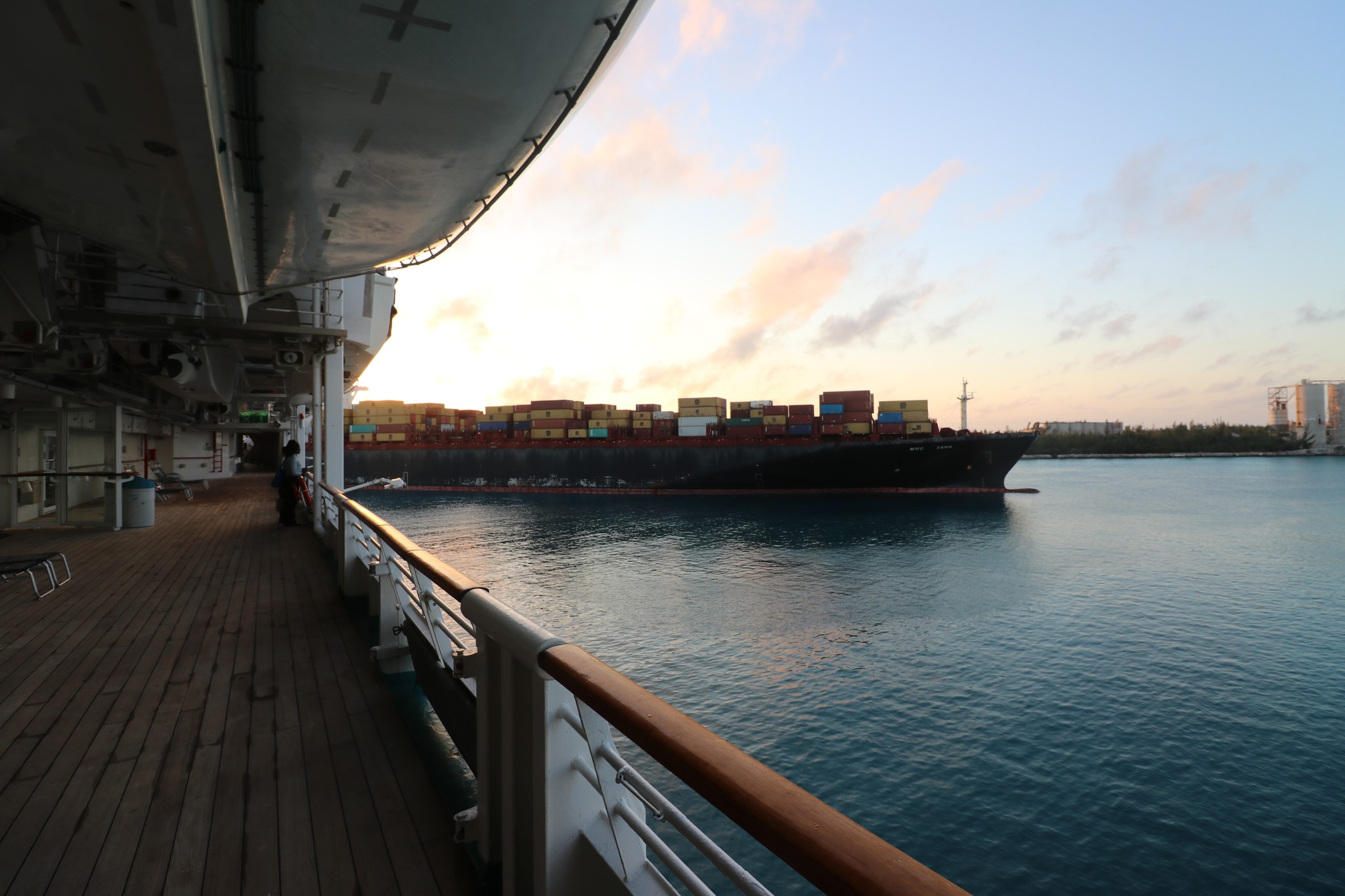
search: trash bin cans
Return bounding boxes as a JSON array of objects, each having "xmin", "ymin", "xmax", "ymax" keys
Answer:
[{"xmin": 104, "ymin": 477, "xmax": 156, "ymax": 527}]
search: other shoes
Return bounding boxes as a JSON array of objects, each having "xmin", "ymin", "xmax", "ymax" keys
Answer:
[{"xmin": 289, "ymin": 522, "xmax": 301, "ymax": 526}]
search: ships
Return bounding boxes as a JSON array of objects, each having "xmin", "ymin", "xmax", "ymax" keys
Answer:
[{"xmin": 344, "ymin": 421, "xmax": 1041, "ymax": 496}]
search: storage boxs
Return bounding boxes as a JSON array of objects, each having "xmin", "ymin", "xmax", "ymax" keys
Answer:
[{"xmin": 322, "ymin": 391, "xmax": 932, "ymax": 442}]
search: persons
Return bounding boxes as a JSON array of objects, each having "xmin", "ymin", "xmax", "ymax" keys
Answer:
[{"xmin": 277, "ymin": 440, "xmax": 310, "ymax": 526}]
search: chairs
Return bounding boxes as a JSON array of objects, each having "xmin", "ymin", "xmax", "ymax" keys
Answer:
[
  {"xmin": 0, "ymin": 552, "xmax": 71, "ymax": 599},
  {"xmin": 124, "ymin": 460, "xmax": 194, "ymax": 502}
]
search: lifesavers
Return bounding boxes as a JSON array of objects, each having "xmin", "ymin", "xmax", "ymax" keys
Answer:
[
  {"xmin": 293, "ymin": 483, "xmax": 301, "ymax": 501},
  {"xmin": 299, "ymin": 475, "xmax": 312, "ymax": 510}
]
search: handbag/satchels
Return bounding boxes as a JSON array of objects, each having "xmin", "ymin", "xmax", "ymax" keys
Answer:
[{"xmin": 270, "ymin": 462, "xmax": 287, "ymax": 489}]
